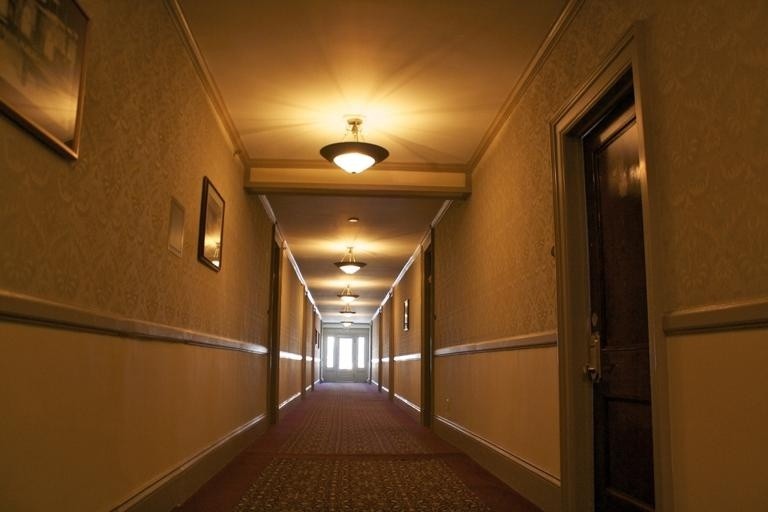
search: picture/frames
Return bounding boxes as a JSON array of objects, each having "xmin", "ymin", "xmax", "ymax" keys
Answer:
[
  {"xmin": 402, "ymin": 299, "xmax": 408, "ymax": 332},
  {"xmin": 198, "ymin": 175, "xmax": 226, "ymax": 273},
  {"xmin": 0, "ymin": 0, "xmax": 91, "ymax": 162}
]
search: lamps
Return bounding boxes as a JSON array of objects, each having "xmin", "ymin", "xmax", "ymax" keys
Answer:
[
  {"xmin": 332, "ymin": 247, "xmax": 366, "ymax": 274},
  {"xmin": 316, "ymin": 115, "xmax": 390, "ymax": 175},
  {"xmin": 335, "ymin": 282, "xmax": 359, "ymax": 328}
]
